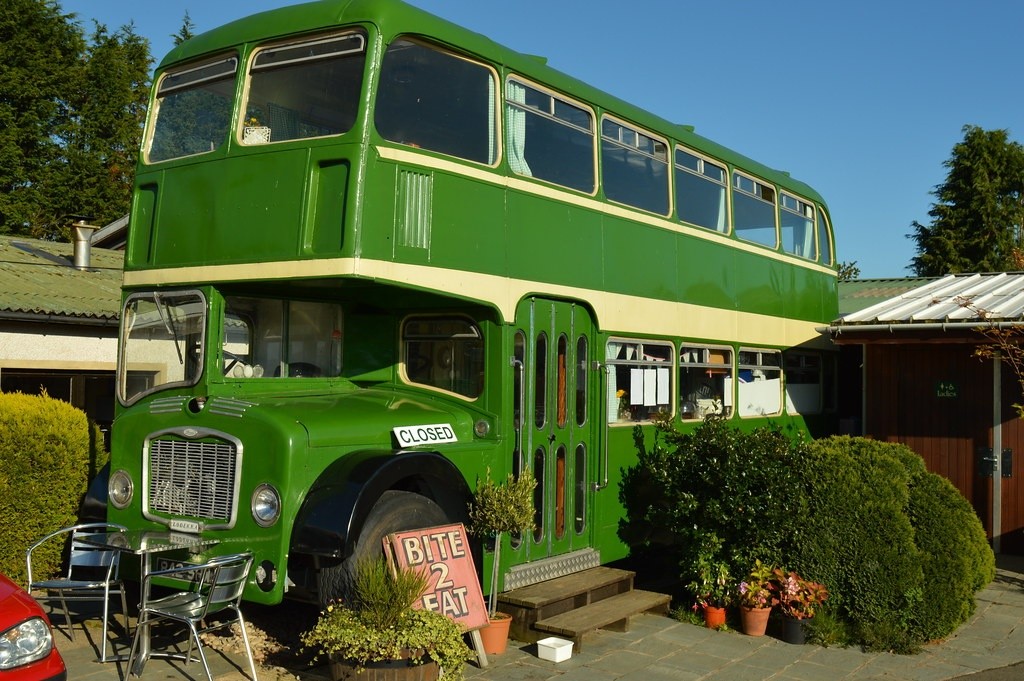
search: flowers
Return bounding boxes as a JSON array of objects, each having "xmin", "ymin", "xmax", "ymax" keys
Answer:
[
  {"xmin": 684, "ymin": 532, "xmax": 827, "ymax": 620},
  {"xmin": 617, "ymin": 388, "xmax": 626, "ymax": 418},
  {"xmin": 245, "ymin": 117, "xmax": 259, "ymax": 126}
]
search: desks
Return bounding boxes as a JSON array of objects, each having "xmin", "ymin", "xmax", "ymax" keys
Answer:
[{"xmin": 73, "ymin": 530, "xmax": 221, "ymax": 678}]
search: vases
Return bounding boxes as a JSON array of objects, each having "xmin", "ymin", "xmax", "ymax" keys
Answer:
[
  {"xmin": 244, "ymin": 126, "xmax": 271, "ymax": 143},
  {"xmin": 765, "ymin": 613, "xmax": 828, "ymax": 643},
  {"xmin": 684, "ymin": 605, "xmax": 737, "ymax": 628},
  {"xmin": 738, "ymin": 609, "xmax": 777, "ymax": 636},
  {"xmin": 617, "ymin": 408, "xmax": 626, "ymax": 423}
]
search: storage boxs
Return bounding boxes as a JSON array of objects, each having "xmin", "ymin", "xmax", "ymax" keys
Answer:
[{"xmin": 537, "ymin": 636, "xmax": 574, "ymax": 662}]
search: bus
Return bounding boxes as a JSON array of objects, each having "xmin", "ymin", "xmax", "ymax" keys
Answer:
[{"xmin": 106, "ymin": 5, "xmax": 844, "ymax": 616}]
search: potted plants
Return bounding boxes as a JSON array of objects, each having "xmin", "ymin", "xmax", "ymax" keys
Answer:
[
  {"xmin": 295, "ymin": 539, "xmax": 482, "ymax": 681},
  {"xmin": 464, "ymin": 462, "xmax": 539, "ymax": 654}
]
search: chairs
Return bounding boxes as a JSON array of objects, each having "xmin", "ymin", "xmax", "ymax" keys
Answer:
[
  {"xmin": 25, "ymin": 522, "xmax": 129, "ymax": 663},
  {"xmin": 122, "ymin": 552, "xmax": 258, "ymax": 681}
]
